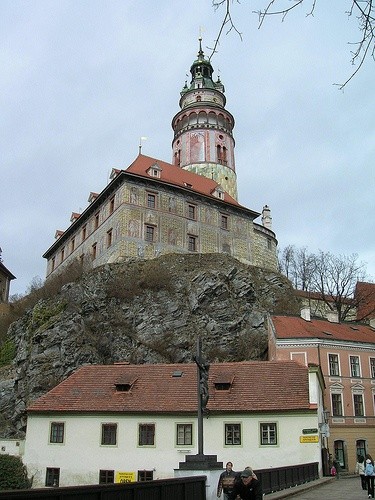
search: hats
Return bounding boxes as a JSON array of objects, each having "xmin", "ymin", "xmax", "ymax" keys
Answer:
[{"xmin": 241, "ymin": 470, "xmax": 252, "ymax": 477}]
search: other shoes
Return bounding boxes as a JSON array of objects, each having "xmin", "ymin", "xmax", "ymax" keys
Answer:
[{"xmin": 368, "ymin": 494, "xmax": 371, "ymax": 499}]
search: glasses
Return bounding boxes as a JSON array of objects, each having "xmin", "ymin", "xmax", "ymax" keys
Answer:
[{"xmin": 242, "ymin": 476, "xmax": 251, "ymax": 479}]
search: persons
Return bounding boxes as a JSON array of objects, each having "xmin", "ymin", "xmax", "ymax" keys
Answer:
[
  {"xmin": 192, "ymin": 355, "xmax": 210, "ymax": 413},
  {"xmin": 328, "ymin": 454, "xmax": 340, "ymax": 476},
  {"xmin": 355, "ymin": 454, "xmax": 375, "ymax": 498},
  {"xmin": 217, "ymin": 462, "xmax": 238, "ymax": 500},
  {"xmin": 228, "ymin": 467, "xmax": 263, "ymax": 500}
]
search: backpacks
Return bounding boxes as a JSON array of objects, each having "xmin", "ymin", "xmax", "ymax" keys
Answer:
[{"xmin": 365, "ymin": 460, "xmax": 374, "ymax": 475}]
